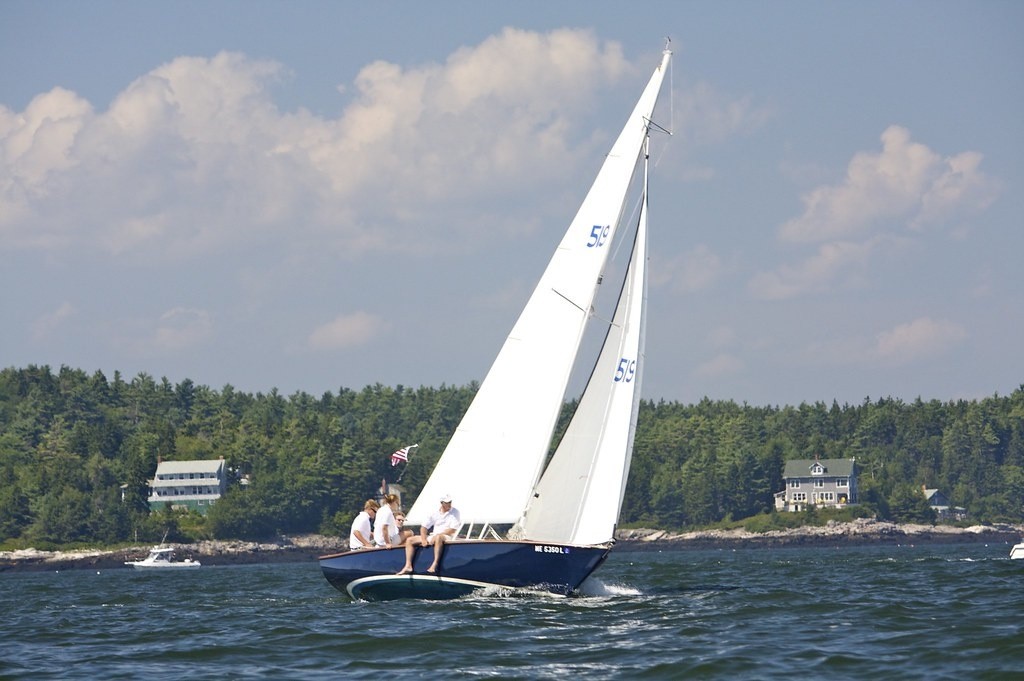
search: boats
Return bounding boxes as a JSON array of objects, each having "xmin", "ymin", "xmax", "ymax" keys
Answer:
[
  {"xmin": 1009, "ymin": 543, "xmax": 1024, "ymax": 560},
  {"xmin": 124, "ymin": 547, "xmax": 201, "ymax": 570}
]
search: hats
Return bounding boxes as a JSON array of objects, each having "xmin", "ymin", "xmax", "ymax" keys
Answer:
[{"xmin": 439, "ymin": 494, "xmax": 452, "ymax": 503}]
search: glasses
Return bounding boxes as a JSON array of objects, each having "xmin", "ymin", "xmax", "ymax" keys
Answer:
[{"xmin": 371, "ymin": 508, "xmax": 376, "ymax": 513}]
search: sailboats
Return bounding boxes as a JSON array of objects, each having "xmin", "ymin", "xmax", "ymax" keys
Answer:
[{"xmin": 320, "ymin": 50, "xmax": 673, "ymax": 600}]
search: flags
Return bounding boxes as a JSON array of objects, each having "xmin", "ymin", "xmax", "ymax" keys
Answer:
[{"xmin": 392, "ymin": 444, "xmax": 418, "ymax": 466}]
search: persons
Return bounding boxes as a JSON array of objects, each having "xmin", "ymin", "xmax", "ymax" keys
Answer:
[
  {"xmin": 350, "ymin": 499, "xmax": 379, "ymax": 551},
  {"xmin": 170, "ymin": 552, "xmax": 177, "ymax": 562},
  {"xmin": 373, "ymin": 494, "xmax": 414, "ymax": 550},
  {"xmin": 396, "ymin": 495, "xmax": 460, "ymax": 575}
]
